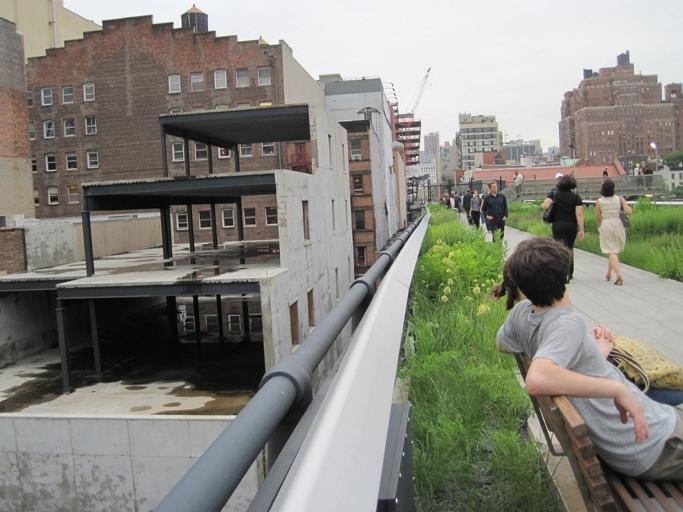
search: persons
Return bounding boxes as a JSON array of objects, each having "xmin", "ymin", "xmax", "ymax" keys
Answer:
[
  {"xmin": 601, "ymin": 165, "xmax": 609, "ymax": 181},
  {"xmin": 592, "ymin": 178, "xmax": 634, "ymax": 286},
  {"xmin": 436, "ymin": 187, "xmax": 493, "ymax": 234},
  {"xmin": 539, "ymin": 174, "xmax": 584, "ymax": 284},
  {"xmin": 631, "ymin": 161, "xmax": 655, "ymax": 192},
  {"xmin": 480, "ymin": 179, "xmax": 508, "ymax": 245},
  {"xmin": 511, "ymin": 170, "xmax": 524, "ymax": 199},
  {"xmin": 492, "ymin": 234, "xmax": 683, "ymax": 486},
  {"xmin": 492, "ymin": 257, "xmax": 683, "ymax": 408}
]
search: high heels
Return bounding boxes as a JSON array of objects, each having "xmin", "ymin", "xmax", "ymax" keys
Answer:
[{"xmin": 605, "ymin": 273, "xmax": 624, "ymax": 285}]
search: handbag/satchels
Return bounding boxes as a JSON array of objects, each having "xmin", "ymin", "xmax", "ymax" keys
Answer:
[
  {"xmin": 610, "ymin": 337, "xmax": 683, "ymax": 394},
  {"xmin": 544, "ymin": 204, "xmax": 554, "ymax": 223},
  {"xmin": 621, "ymin": 213, "xmax": 630, "ymax": 227}
]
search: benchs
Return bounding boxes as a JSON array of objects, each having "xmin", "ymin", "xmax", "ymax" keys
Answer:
[{"xmin": 514, "ymin": 351, "xmax": 683, "ymax": 512}]
